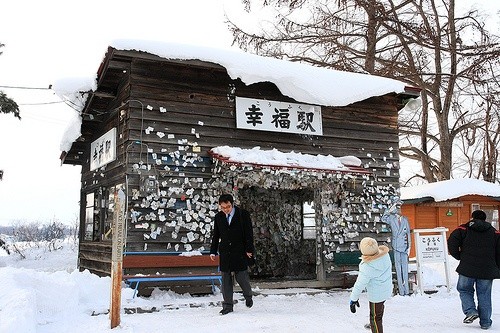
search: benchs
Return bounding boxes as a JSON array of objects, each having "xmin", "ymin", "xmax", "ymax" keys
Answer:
[
  {"xmin": 123, "ymin": 251, "xmax": 223, "ymax": 299},
  {"xmin": 334, "ymin": 251, "xmax": 417, "ymax": 296}
]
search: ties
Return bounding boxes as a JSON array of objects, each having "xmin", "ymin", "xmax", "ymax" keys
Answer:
[{"xmin": 227, "ymin": 214, "xmax": 230, "ymax": 222}]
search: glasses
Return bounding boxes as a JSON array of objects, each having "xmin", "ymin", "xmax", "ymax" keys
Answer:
[{"xmin": 222, "ymin": 205, "xmax": 231, "ymax": 209}]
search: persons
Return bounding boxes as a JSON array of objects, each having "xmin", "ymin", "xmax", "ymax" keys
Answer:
[
  {"xmin": 350, "ymin": 237, "xmax": 392, "ymax": 333},
  {"xmin": 447, "ymin": 210, "xmax": 500, "ymax": 330},
  {"xmin": 210, "ymin": 194, "xmax": 255, "ymax": 316}
]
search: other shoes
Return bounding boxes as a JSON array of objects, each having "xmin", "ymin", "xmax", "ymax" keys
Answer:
[
  {"xmin": 220, "ymin": 310, "xmax": 233, "ymax": 315},
  {"xmin": 246, "ymin": 299, "xmax": 253, "ymax": 308},
  {"xmin": 464, "ymin": 315, "xmax": 478, "ymax": 323},
  {"xmin": 481, "ymin": 320, "xmax": 492, "ymax": 329}
]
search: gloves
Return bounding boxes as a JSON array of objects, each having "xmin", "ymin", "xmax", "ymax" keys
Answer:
[{"xmin": 350, "ymin": 300, "xmax": 360, "ymax": 313}]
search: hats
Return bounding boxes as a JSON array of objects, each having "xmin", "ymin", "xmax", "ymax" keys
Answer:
[
  {"xmin": 472, "ymin": 210, "xmax": 487, "ymax": 220},
  {"xmin": 361, "ymin": 238, "xmax": 377, "ymax": 255}
]
